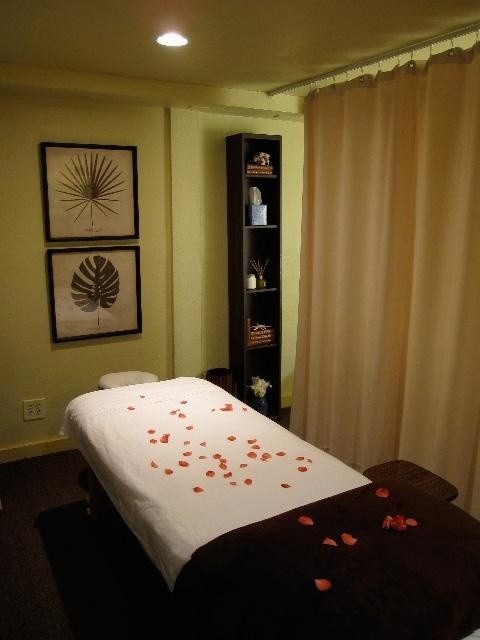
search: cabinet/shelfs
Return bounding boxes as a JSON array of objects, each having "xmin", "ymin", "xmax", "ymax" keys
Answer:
[{"xmin": 224, "ymin": 131, "xmax": 283, "ymax": 421}]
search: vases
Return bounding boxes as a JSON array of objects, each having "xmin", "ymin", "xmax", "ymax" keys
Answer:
[{"xmin": 252, "ymin": 397, "xmax": 269, "ymax": 416}]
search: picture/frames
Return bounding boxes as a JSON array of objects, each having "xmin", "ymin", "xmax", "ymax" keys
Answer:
[{"xmin": 39, "ymin": 141, "xmax": 144, "ymax": 345}]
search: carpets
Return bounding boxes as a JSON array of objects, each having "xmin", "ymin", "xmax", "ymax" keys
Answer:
[{"xmin": 30, "ymin": 498, "xmax": 224, "ymax": 640}]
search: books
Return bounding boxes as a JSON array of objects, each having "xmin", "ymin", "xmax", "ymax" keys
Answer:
[
  {"xmin": 246, "ymin": 163, "xmax": 273, "ymax": 175},
  {"xmin": 244, "ymin": 318, "xmax": 276, "ymax": 348}
]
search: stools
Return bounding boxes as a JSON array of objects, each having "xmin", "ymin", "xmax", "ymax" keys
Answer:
[
  {"xmin": 97, "ymin": 371, "xmax": 158, "ymax": 390},
  {"xmin": 361, "ymin": 459, "xmax": 458, "ymax": 502}
]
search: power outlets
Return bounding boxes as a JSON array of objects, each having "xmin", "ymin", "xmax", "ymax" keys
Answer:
[{"xmin": 23, "ymin": 397, "xmax": 48, "ymax": 421}]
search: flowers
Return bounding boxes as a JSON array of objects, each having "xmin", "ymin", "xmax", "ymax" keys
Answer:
[{"xmin": 247, "ymin": 374, "xmax": 274, "ymax": 398}]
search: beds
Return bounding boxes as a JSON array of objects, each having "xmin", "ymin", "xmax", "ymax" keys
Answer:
[{"xmin": 67, "ymin": 376, "xmax": 477, "ymax": 640}]
